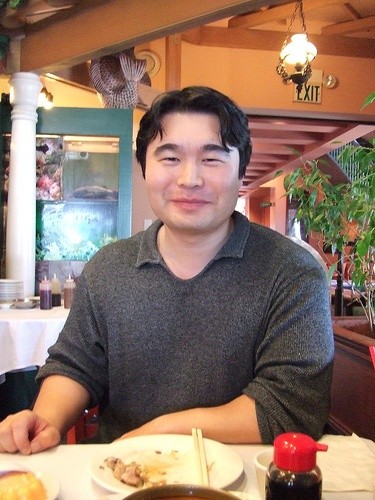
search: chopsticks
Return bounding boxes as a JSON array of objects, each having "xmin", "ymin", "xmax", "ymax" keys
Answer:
[{"xmin": 191, "ymin": 427, "xmax": 209, "ymax": 488}]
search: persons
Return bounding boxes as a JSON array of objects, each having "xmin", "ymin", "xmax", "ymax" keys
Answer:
[{"xmin": 0, "ymin": 85, "xmax": 337, "ymax": 456}]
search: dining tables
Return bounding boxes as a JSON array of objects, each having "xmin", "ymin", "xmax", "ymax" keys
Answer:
[{"xmin": 0, "ymin": 442, "xmax": 375, "ymax": 500}]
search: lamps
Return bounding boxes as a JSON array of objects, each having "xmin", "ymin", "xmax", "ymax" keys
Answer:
[
  {"xmin": 274, "ymin": 0, "xmax": 319, "ymax": 93},
  {"xmin": 38, "ymin": 86, "xmax": 54, "ymax": 110},
  {"xmin": 282, "ymin": 68, "xmax": 337, "ymax": 105}
]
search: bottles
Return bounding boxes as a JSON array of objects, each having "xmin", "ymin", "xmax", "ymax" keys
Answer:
[
  {"xmin": 39, "ymin": 275, "xmax": 53, "ymax": 309},
  {"xmin": 264, "ymin": 433, "xmax": 329, "ymax": 499},
  {"xmin": 50, "ymin": 273, "xmax": 62, "ymax": 306},
  {"xmin": 63, "ymin": 274, "xmax": 76, "ymax": 309}
]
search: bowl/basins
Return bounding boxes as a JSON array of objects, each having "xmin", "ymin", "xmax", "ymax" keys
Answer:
[
  {"xmin": 123, "ymin": 484, "xmax": 241, "ymax": 499},
  {"xmin": 12, "ymin": 296, "xmax": 40, "ymax": 309}
]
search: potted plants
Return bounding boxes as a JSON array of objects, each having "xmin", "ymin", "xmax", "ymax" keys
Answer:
[{"xmin": 273, "ymin": 89, "xmax": 375, "ymax": 442}]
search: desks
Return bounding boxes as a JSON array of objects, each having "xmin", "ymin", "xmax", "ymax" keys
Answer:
[{"xmin": 0, "ymin": 298, "xmax": 71, "ymax": 382}]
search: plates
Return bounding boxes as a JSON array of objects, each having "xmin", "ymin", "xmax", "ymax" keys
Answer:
[
  {"xmin": 0, "ymin": 451, "xmax": 60, "ymax": 500},
  {"xmin": 89, "ymin": 432, "xmax": 245, "ymax": 494},
  {"xmin": 0, "ymin": 278, "xmax": 25, "ymax": 302}
]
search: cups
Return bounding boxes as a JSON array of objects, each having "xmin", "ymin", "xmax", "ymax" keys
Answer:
[{"xmin": 255, "ymin": 451, "xmax": 273, "ymax": 500}]
